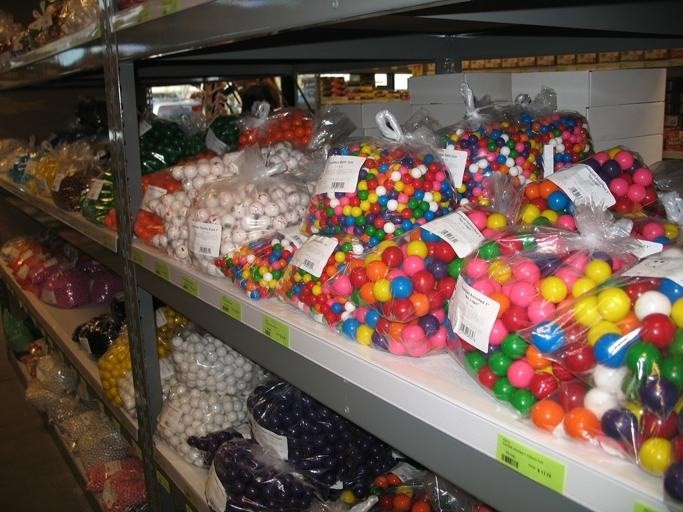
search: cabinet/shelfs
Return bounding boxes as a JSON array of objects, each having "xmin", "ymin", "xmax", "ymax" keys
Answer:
[{"xmin": 1, "ymin": 1, "xmax": 683, "ymax": 512}]
